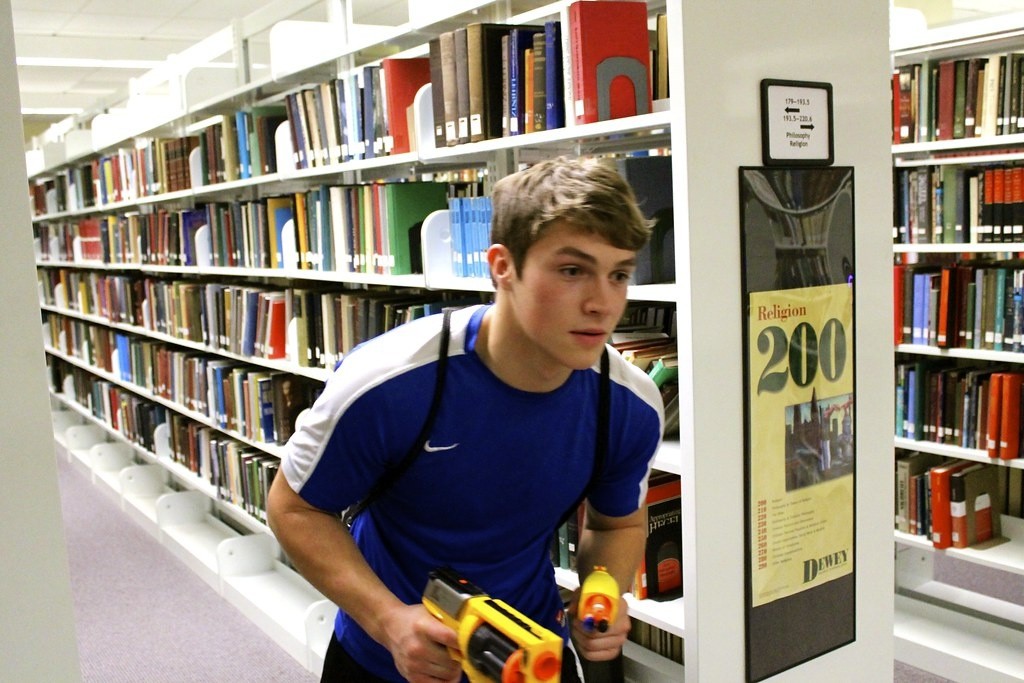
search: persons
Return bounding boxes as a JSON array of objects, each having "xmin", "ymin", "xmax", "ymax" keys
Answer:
[{"xmin": 265, "ymin": 154, "xmax": 666, "ymax": 683}]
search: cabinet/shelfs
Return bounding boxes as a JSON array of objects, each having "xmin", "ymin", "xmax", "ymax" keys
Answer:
[
  {"xmin": 889, "ymin": 25, "xmax": 1024, "ymax": 683},
  {"xmin": 27, "ymin": 0, "xmax": 895, "ymax": 683}
]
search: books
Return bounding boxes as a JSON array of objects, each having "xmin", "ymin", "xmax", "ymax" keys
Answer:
[
  {"xmin": 891, "ymin": 54, "xmax": 1024, "ymax": 551},
  {"xmin": 26, "ymin": 2, "xmax": 684, "ymax": 666}
]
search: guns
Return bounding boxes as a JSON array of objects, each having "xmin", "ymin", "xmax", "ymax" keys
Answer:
[
  {"xmin": 577, "ymin": 565, "xmax": 621, "ymax": 633},
  {"xmin": 422, "ymin": 569, "xmax": 562, "ymax": 683}
]
